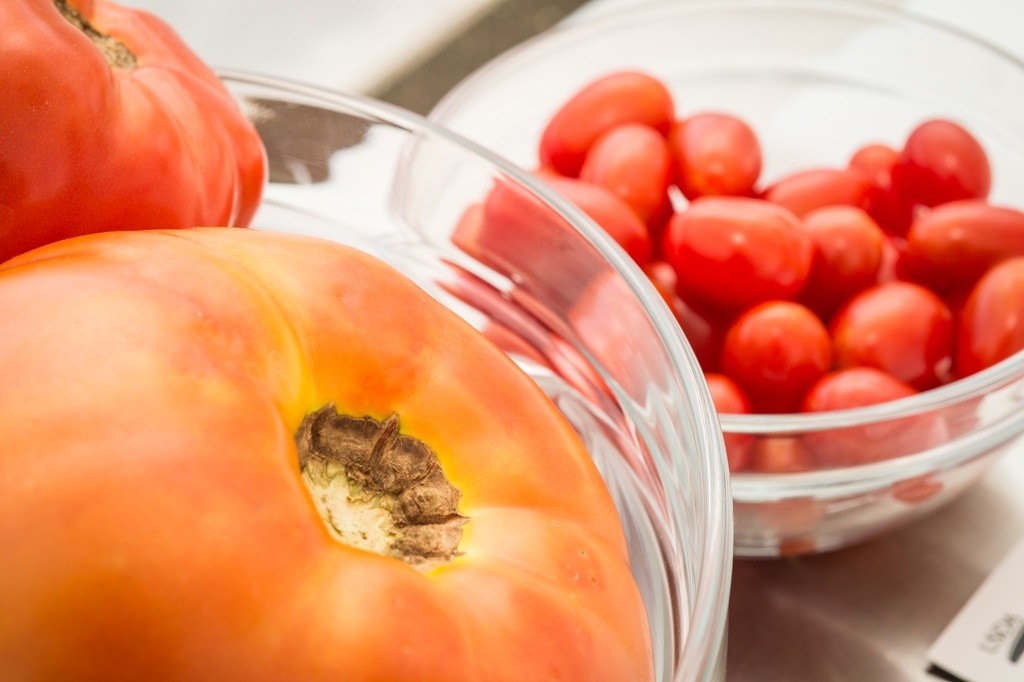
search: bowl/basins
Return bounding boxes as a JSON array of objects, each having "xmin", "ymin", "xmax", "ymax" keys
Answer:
[
  {"xmin": 386, "ymin": 0, "xmax": 1024, "ymax": 557},
  {"xmin": 207, "ymin": 65, "xmax": 736, "ymax": 682}
]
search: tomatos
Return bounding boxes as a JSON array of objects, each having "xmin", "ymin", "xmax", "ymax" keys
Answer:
[
  {"xmin": 0, "ymin": 226, "xmax": 654, "ymax": 682},
  {"xmin": 436, "ymin": 71, "xmax": 1024, "ymax": 554},
  {"xmin": 0, "ymin": 0, "xmax": 269, "ymax": 263}
]
